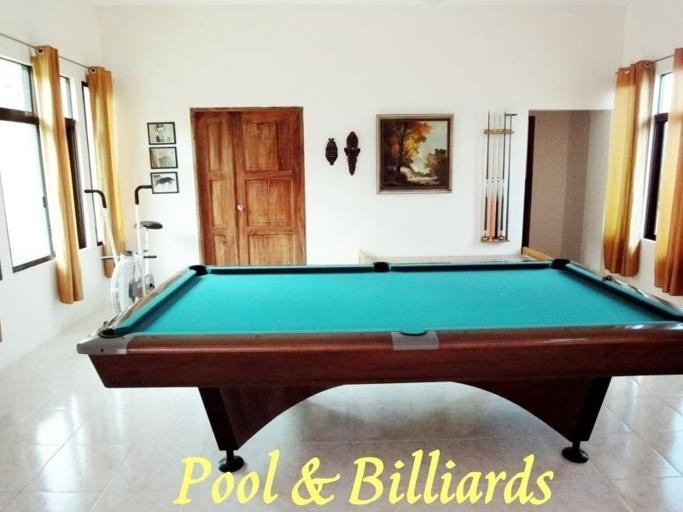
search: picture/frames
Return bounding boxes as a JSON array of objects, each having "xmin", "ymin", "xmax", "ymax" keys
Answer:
[
  {"xmin": 145, "ymin": 121, "xmax": 179, "ymax": 195},
  {"xmin": 375, "ymin": 113, "xmax": 455, "ymax": 193}
]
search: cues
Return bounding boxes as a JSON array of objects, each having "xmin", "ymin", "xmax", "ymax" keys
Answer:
[{"xmin": 483, "ymin": 112, "xmax": 512, "ymax": 242}]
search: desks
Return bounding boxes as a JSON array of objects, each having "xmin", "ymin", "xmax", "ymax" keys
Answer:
[{"xmin": 76, "ymin": 256, "xmax": 683, "ymax": 473}]
